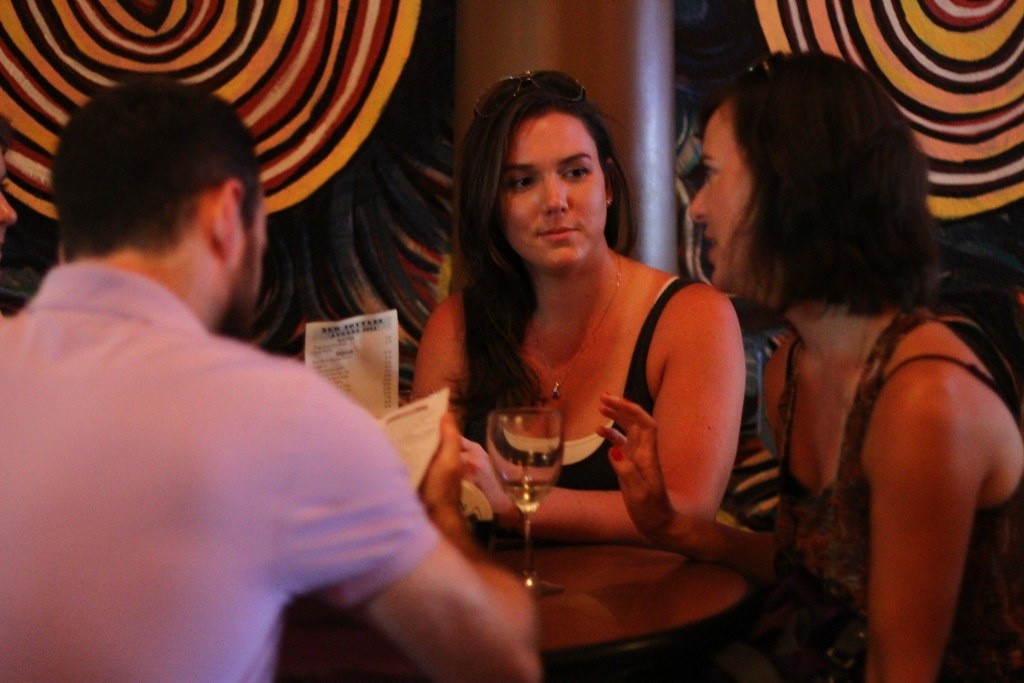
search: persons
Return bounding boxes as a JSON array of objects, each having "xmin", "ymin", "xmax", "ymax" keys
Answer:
[
  {"xmin": 410, "ymin": 70, "xmax": 746, "ymax": 546},
  {"xmin": 595, "ymin": 52, "xmax": 1024, "ymax": 683},
  {"xmin": 0, "ymin": 83, "xmax": 543, "ymax": 683}
]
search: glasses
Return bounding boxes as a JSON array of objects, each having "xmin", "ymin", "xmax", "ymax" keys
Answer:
[
  {"xmin": 742, "ymin": 50, "xmax": 787, "ymax": 86},
  {"xmin": 471, "ymin": 69, "xmax": 589, "ymax": 121}
]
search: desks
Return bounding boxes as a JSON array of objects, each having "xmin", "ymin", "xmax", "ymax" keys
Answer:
[{"xmin": 275, "ymin": 538, "xmax": 752, "ymax": 682}]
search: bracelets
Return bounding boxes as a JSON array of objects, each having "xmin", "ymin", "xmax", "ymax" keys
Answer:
[{"xmin": 424, "ymin": 498, "xmax": 460, "ymax": 510}]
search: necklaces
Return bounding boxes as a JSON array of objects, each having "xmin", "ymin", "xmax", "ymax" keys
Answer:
[
  {"xmin": 526, "ymin": 251, "xmax": 622, "ymax": 399},
  {"xmin": 797, "ymin": 315, "xmax": 871, "ymax": 466}
]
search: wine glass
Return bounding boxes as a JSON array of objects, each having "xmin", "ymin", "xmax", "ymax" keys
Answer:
[{"xmin": 487, "ymin": 408, "xmax": 565, "ymax": 599}]
